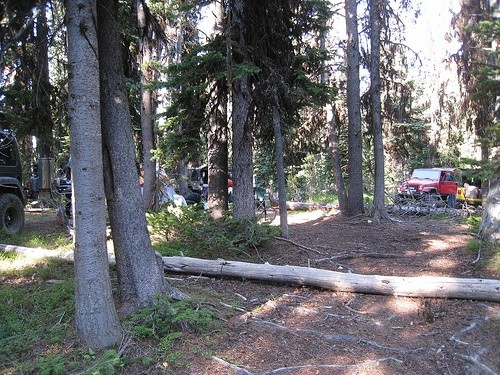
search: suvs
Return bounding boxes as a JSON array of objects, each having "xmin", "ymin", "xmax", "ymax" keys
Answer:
[
  {"xmin": 394, "ymin": 167, "xmax": 462, "ymax": 209},
  {"xmin": 0, "ymin": 131, "xmax": 28, "ymax": 238}
]
